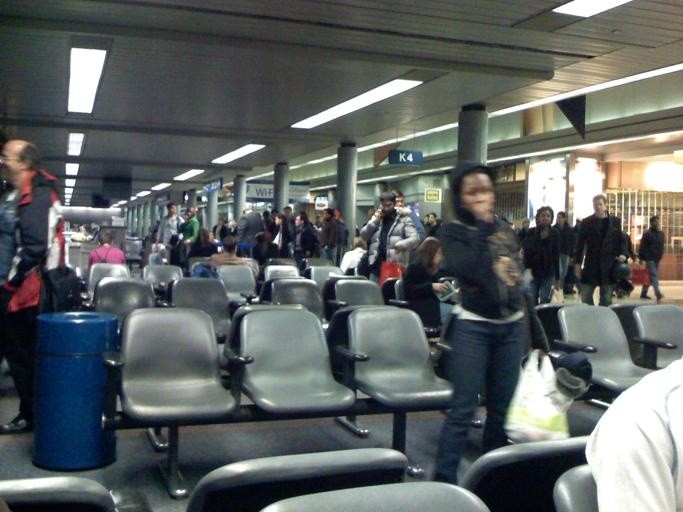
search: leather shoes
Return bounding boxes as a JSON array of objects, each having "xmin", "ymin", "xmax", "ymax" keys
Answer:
[{"xmin": 1, "ymin": 412, "xmax": 29, "ymax": 434}]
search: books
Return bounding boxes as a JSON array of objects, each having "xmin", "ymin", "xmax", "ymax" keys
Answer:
[{"xmin": 435, "ymin": 281, "xmax": 459, "ymax": 301}]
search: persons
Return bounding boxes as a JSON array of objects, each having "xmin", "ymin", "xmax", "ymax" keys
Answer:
[
  {"xmin": 1, "ymin": 141, "xmax": 69, "ymax": 434},
  {"xmin": 526, "ymin": 206, "xmax": 561, "ymax": 306},
  {"xmin": 261, "ymin": 211, "xmax": 276, "ymax": 235},
  {"xmin": 213, "ymin": 218, "xmax": 229, "ymax": 237},
  {"xmin": 284, "ymin": 206, "xmax": 295, "ymax": 237},
  {"xmin": 339, "ymin": 237, "xmax": 368, "ymax": 275},
  {"xmin": 424, "ymin": 214, "xmax": 439, "ymax": 233},
  {"xmin": 515, "ymin": 220, "xmax": 530, "ymax": 241},
  {"xmin": 209, "ymin": 237, "xmax": 247, "ymax": 267},
  {"xmin": 236, "ymin": 202, "xmax": 265, "ymax": 267},
  {"xmin": 572, "ymin": 195, "xmax": 624, "ymax": 306},
  {"xmin": 585, "ymin": 357, "xmax": 682, "ymax": 512},
  {"xmin": 638, "ymin": 216, "xmax": 665, "ymax": 300},
  {"xmin": 551, "ymin": 213, "xmax": 573, "ymax": 294},
  {"xmin": 154, "ymin": 203, "xmax": 186, "ymax": 266},
  {"xmin": 404, "ymin": 237, "xmax": 446, "ymax": 347},
  {"xmin": 270, "ymin": 215, "xmax": 288, "ymax": 254},
  {"xmin": 390, "ymin": 192, "xmax": 424, "ymax": 277},
  {"xmin": 433, "ymin": 159, "xmax": 530, "ymax": 483},
  {"xmin": 88, "ymin": 231, "xmax": 126, "ymax": 265},
  {"xmin": 318, "ymin": 210, "xmax": 337, "ymax": 263},
  {"xmin": 361, "ymin": 190, "xmax": 418, "ymax": 283},
  {"xmin": 614, "ymin": 225, "xmax": 636, "ymax": 298},
  {"xmin": 188, "ymin": 229, "xmax": 218, "ymax": 262},
  {"xmin": 175, "ymin": 206, "xmax": 200, "ymax": 264},
  {"xmin": 313, "ymin": 215, "xmax": 323, "ymax": 233},
  {"xmin": 291, "ymin": 214, "xmax": 312, "ymax": 268}
]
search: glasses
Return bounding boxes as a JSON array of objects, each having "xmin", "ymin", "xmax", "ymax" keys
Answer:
[{"xmin": 0, "ymin": 156, "xmax": 18, "ymax": 163}]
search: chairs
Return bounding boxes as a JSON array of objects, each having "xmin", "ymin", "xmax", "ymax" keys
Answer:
[
  {"xmin": 142, "ymin": 265, "xmax": 184, "ymax": 298},
  {"xmin": 335, "ymin": 280, "xmax": 384, "ymax": 316},
  {"xmin": 272, "ymin": 279, "xmax": 324, "ymax": 320},
  {"xmin": 264, "ymin": 265, "xmax": 300, "ymax": 288},
  {"xmin": 215, "ymin": 265, "xmax": 257, "ymax": 304},
  {"xmin": 230, "ymin": 308, "xmax": 356, "ymax": 419},
  {"xmin": 92, "ymin": 276, "xmax": 156, "ymax": 325},
  {"xmin": 305, "ymin": 266, "xmax": 344, "ymax": 290},
  {"xmin": 101, "ymin": 304, "xmax": 236, "ymax": 422},
  {"xmin": 553, "ymin": 304, "xmax": 655, "ymax": 402},
  {"xmin": 343, "ymin": 305, "xmax": 458, "ymax": 412},
  {"xmin": 632, "ymin": 303, "xmax": 683, "ymax": 368},
  {"xmin": 169, "ymin": 277, "xmax": 235, "ymax": 344}
]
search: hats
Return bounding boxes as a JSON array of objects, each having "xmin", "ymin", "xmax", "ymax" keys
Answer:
[{"xmin": 447, "ymin": 161, "xmax": 506, "ymax": 189}]
